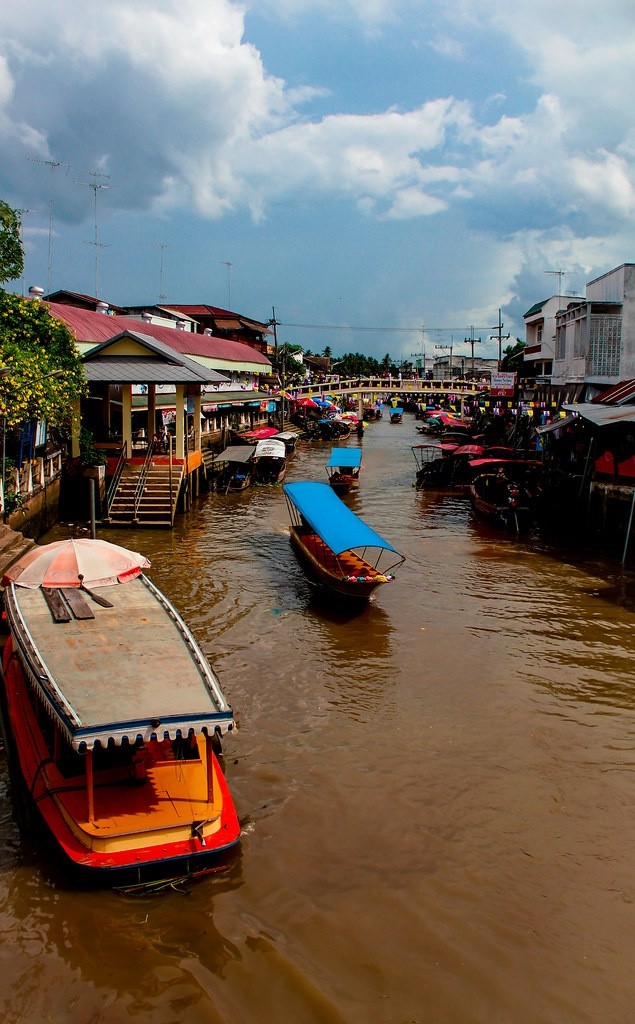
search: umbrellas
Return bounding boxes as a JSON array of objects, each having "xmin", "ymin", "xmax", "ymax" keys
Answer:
[
  {"xmin": 4, "ymin": 538, "xmax": 153, "ymax": 589},
  {"xmin": 271, "ymin": 390, "xmax": 357, "ymax": 417}
]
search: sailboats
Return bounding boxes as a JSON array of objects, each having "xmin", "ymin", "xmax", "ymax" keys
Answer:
[{"xmin": 233, "ymin": 427, "xmax": 281, "ymax": 448}]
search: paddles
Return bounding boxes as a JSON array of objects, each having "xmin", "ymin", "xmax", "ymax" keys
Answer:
[{"xmin": 82, "ymin": 585, "xmax": 114, "ymax": 608}]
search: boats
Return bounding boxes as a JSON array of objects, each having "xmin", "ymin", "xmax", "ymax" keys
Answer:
[
  {"xmin": 389, "ymin": 407, "xmax": 403, "ymax": 424},
  {"xmin": 266, "ymin": 431, "xmax": 299, "ymax": 462},
  {"xmin": 324, "ymin": 446, "xmax": 363, "ymax": 494},
  {"xmin": 282, "ymin": 480, "xmax": 407, "ymax": 603},
  {"xmin": 1, "ymin": 538, "xmax": 243, "ymax": 899},
  {"xmin": 302, "ymin": 391, "xmax": 635, "ymax": 533},
  {"xmin": 210, "ymin": 443, "xmax": 257, "ymax": 494},
  {"xmin": 251, "ymin": 438, "xmax": 288, "ymax": 485}
]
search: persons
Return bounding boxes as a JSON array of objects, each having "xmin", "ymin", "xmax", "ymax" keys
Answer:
[
  {"xmin": 161, "ymin": 429, "xmax": 169, "ymax": 454},
  {"xmin": 261, "ymin": 371, "xmax": 489, "ymax": 390},
  {"xmin": 185, "ymin": 416, "xmax": 248, "ymax": 438}
]
